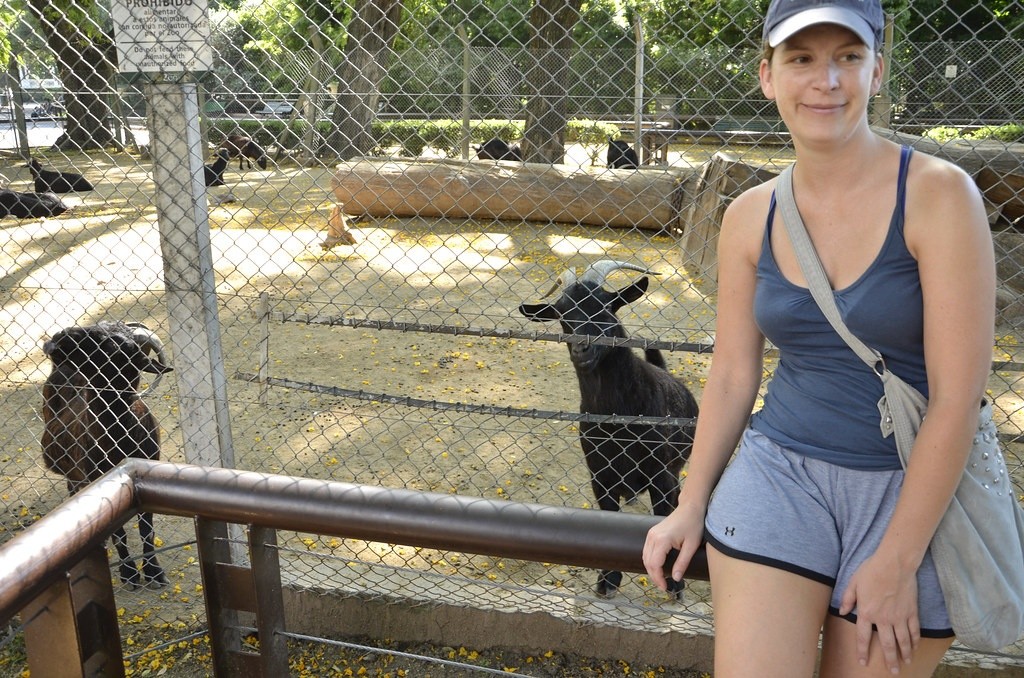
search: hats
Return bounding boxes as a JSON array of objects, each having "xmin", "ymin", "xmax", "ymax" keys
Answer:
[{"xmin": 761, "ymin": 0, "xmax": 884, "ymax": 51}]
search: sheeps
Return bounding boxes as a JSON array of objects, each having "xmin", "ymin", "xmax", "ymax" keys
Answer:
[
  {"xmin": 220, "ymin": 134, "xmax": 267, "ymax": 170},
  {"xmin": 606, "ymin": 137, "xmax": 638, "ymax": 169},
  {"xmin": 518, "ymin": 260, "xmax": 700, "ymax": 596},
  {"xmin": 43, "ymin": 319, "xmax": 178, "ymax": 591},
  {"xmin": 472, "ymin": 139, "xmax": 521, "ymax": 161},
  {"xmin": 0, "ymin": 173, "xmax": 68, "ymax": 219},
  {"xmin": 204, "ymin": 148, "xmax": 230, "ymax": 187},
  {"xmin": 28, "ymin": 157, "xmax": 95, "ymax": 193}
]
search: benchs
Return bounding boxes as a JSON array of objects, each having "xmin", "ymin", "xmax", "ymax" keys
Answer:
[
  {"xmin": 714, "ymin": 116, "xmax": 789, "ymax": 140},
  {"xmin": 205, "ymin": 102, "xmax": 228, "ymax": 115},
  {"xmin": 255, "ymin": 103, "xmax": 296, "ymax": 118}
]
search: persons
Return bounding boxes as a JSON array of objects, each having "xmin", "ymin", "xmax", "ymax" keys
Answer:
[{"xmin": 640, "ymin": 0, "xmax": 994, "ymax": 678}]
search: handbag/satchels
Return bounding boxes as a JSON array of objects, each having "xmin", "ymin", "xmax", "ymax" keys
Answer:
[{"xmin": 776, "ymin": 164, "xmax": 1024, "ymax": 649}]
